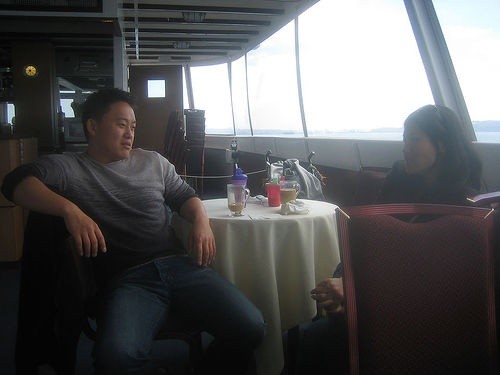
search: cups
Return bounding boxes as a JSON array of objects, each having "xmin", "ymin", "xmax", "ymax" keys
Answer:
[
  {"xmin": 278, "ymin": 179, "xmax": 301, "ymax": 208},
  {"xmin": 227, "ymin": 183, "xmax": 250, "ymax": 216},
  {"xmin": 266, "ymin": 183, "xmax": 281, "ymax": 207}
]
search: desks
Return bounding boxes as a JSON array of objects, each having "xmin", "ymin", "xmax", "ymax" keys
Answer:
[{"xmin": 173, "ymin": 199, "xmax": 341, "ymax": 375}]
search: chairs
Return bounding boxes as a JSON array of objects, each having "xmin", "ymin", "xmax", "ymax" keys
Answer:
[
  {"xmin": 15, "ymin": 185, "xmax": 203, "ymax": 375},
  {"xmin": 335, "ymin": 203, "xmax": 500, "ymax": 375}
]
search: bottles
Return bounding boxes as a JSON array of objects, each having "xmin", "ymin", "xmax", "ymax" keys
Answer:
[{"xmin": 231, "ymin": 168, "xmax": 248, "ymax": 209}]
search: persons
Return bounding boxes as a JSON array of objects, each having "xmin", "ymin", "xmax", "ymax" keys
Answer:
[
  {"xmin": 280, "ymin": 105, "xmax": 482, "ymax": 375},
  {"xmin": 2, "ymin": 88, "xmax": 265, "ymax": 375}
]
